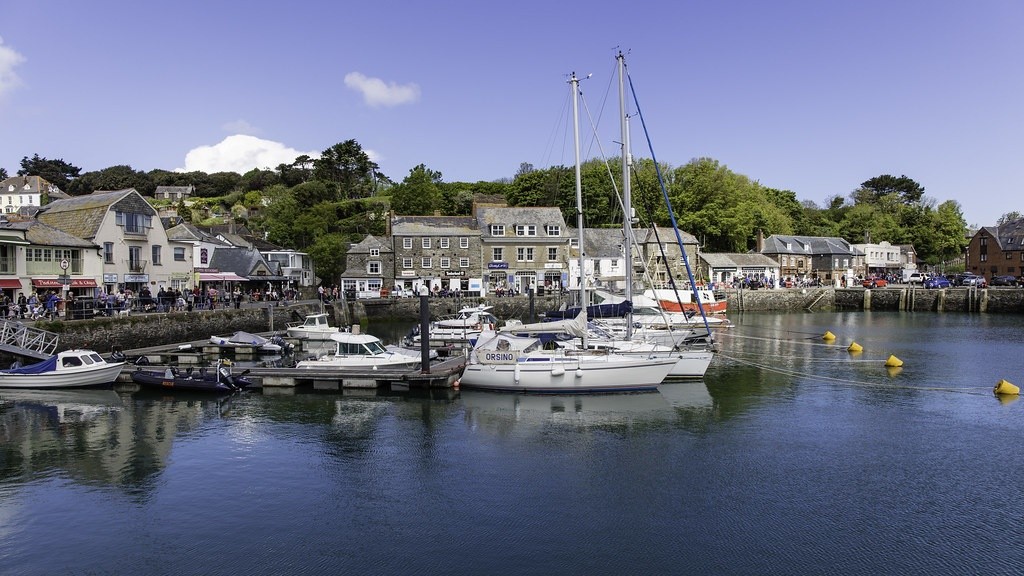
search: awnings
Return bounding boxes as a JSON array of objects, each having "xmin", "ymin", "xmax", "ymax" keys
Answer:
[
  {"xmin": 32, "ymin": 279, "xmax": 98, "ymax": 288},
  {"xmin": 0, "ymin": 279, "xmax": 22, "ymax": 289},
  {"xmin": 199, "ymin": 274, "xmax": 289, "ymax": 282}
]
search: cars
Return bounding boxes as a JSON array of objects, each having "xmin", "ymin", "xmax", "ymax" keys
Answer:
[
  {"xmin": 990, "ymin": 275, "xmax": 996, "ymax": 285},
  {"xmin": 1014, "ymin": 276, "xmax": 1024, "ymax": 287},
  {"xmin": 947, "ymin": 272, "xmax": 973, "ymax": 286},
  {"xmin": 994, "ymin": 275, "xmax": 1017, "ymax": 286},
  {"xmin": 963, "ymin": 275, "xmax": 986, "ymax": 287},
  {"xmin": 923, "ymin": 276, "xmax": 951, "ymax": 289},
  {"xmin": 862, "ymin": 278, "xmax": 889, "ymax": 288}
]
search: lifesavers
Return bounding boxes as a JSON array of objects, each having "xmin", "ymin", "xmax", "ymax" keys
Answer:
[
  {"xmin": 489, "ymin": 323, "xmax": 493, "ymax": 331},
  {"xmin": 475, "ymin": 322, "xmax": 482, "ymax": 330}
]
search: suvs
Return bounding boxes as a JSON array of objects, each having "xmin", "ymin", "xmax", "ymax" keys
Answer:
[{"xmin": 909, "ymin": 272, "xmax": 930, "ymax": 283}]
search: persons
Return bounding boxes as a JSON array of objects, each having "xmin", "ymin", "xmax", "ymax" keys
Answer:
[
  {"xmin": 0, "ymin": 284, "xmax": 301, "ymax": 321},
  {"xmin": 732, "ymin": 273, "xmax": 1024, "ymax": 290},
  {"xmin": 316, "ymin": 280, "xmax": 567, "ymax": 301}
]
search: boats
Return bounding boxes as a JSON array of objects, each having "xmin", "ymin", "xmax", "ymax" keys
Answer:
[
  {"xmin": 172, "ymin": 47, "xmax": 730, "ymax": 389},
  {"xmin": 0, "ymin": 350, "xmax": 125, "ymax": 387},
  {"xmin": 132, "ymin": 357, "xmax": 253, "ymax": 394}
]
